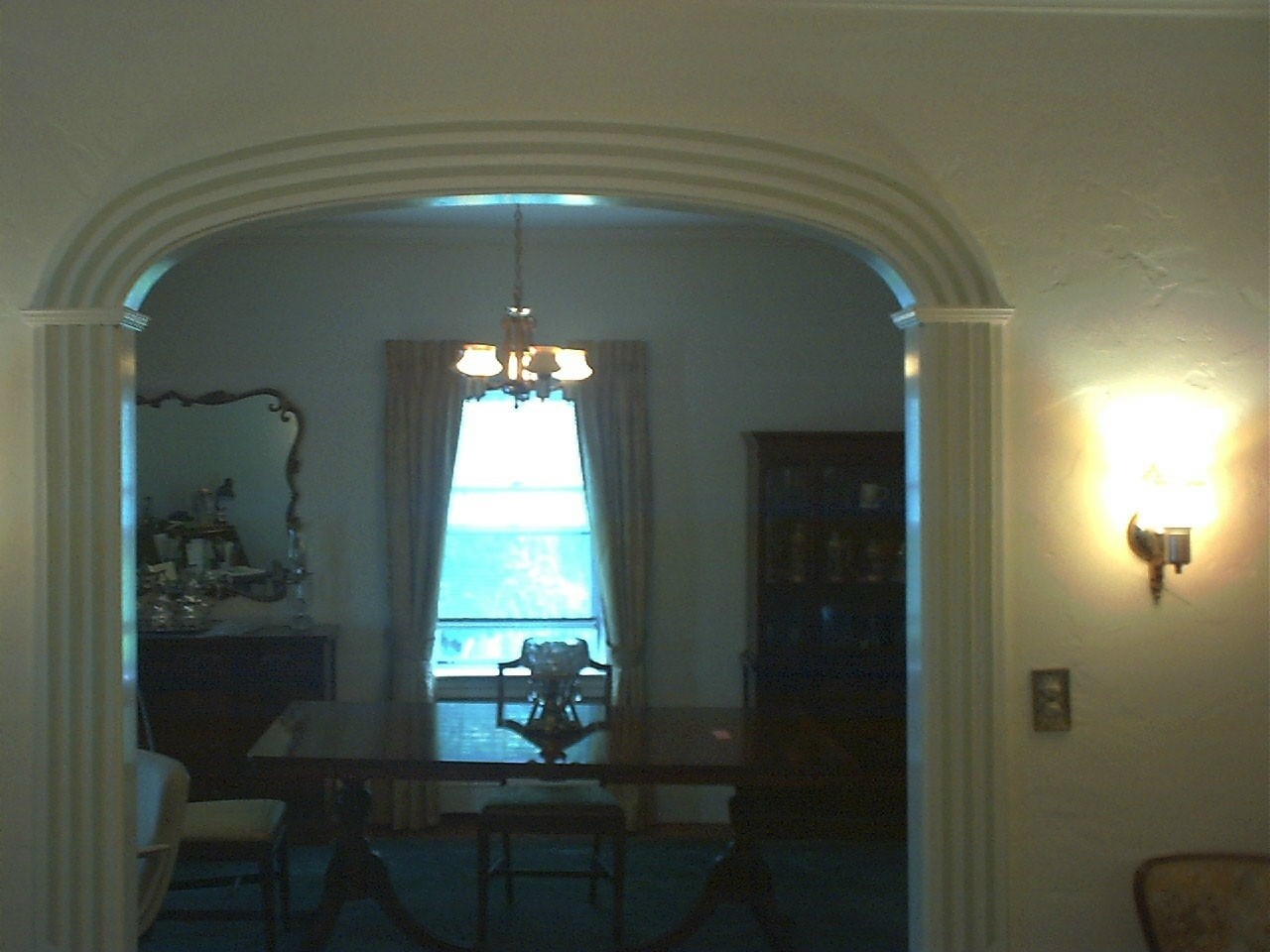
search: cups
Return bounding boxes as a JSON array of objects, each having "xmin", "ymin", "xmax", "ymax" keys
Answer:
[{"xmin": 857, "ymin": 483, "xmax": 891, "ymax": 509}]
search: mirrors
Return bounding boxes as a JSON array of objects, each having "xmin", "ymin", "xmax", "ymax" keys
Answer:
[{"xmin": 137, "ymin": 388, "xmax": 308, "ymax": 602}]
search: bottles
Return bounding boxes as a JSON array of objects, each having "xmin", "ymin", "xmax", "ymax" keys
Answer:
[
  {"xmin": 856, "ymin": 546, "xmax": 894, "ymax": 583},
  {"xmin": 789, "ymin": 522, "xmax": 808, "ymax": 584},
  {"xmin": 826, "ymin": 526, "xmax": 848, "ymax": 584}
]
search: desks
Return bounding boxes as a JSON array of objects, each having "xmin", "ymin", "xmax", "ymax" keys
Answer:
[{"xmin": 246, "ymin": 698, "xmax": 890, "ymax": 952}]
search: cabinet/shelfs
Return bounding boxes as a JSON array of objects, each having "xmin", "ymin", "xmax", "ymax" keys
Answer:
[
  {"xmin": 139, "ymin": 626, "xmax": 335, "ymax": 800},
  {"xmin": 740, "ymin": 429, "xmax": 907, "ymax": 840}
]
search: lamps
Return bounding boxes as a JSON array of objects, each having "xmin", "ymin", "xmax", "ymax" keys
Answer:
[
  {"xmin": 1092, "ymin": 392, "xmax": 1234, "ymax": 574},
  {"xmin": 455, "ymin": 206, "xmax": 593, "ymax": 407}
]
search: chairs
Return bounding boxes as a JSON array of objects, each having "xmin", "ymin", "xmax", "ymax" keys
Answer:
[
  {"xmin": 1134, "ymin": 853, "xmax": 1270, "ymax": 952},
  {"xmin": 140, "ymin": 691, "xmax": 291, "ymax": 952},
  {"xmin": 474, "ymin": 635, "xmax": 626, "ymax": 943}
]
